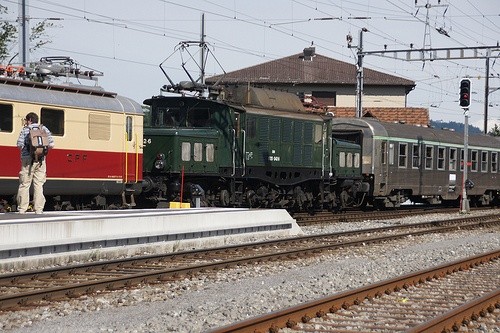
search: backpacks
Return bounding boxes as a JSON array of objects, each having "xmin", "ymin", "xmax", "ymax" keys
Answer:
[{"xmin": 25, "ymin": 124, "xmax": 48, "ymax": 167}]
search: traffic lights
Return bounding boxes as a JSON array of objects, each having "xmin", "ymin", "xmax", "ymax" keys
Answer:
[{"xmin": 459, "ymin": 78, "xmax": 471, "ymax": 109}]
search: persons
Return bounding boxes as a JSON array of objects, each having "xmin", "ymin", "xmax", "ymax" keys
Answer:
[{"xmin": 17, "ymin": 111, "xmax": 54, "ymax": 215}]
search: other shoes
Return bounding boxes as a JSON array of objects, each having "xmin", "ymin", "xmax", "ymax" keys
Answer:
[
  {"xmin": 19, "ymin": 210, "xmax": 26, "ymax": 215},
  {"xmin": 35, "ymin": 210, "xmax": 43, "ymax": 214}
]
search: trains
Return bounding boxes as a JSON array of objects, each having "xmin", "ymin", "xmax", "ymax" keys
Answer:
[{"xmin": 0, "ymin": 40, "xmax": 500, "ymax": 215}]
